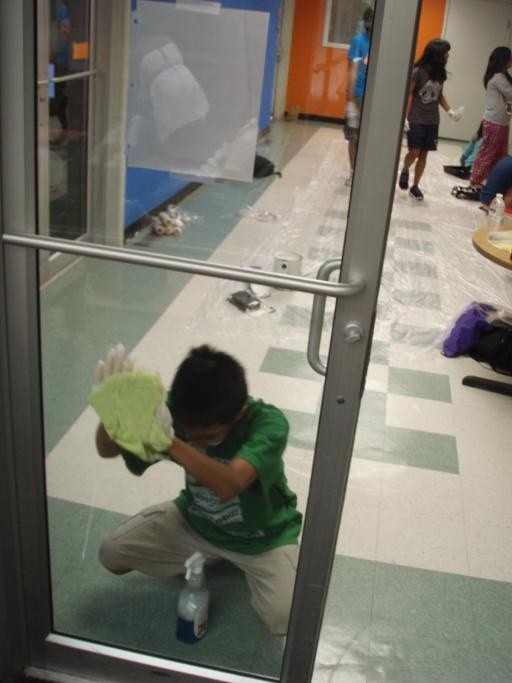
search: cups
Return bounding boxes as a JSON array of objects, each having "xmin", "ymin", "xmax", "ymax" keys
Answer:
[{"xmin": 272, "ymin": 250, "xmax": 303, "ymax": 276}]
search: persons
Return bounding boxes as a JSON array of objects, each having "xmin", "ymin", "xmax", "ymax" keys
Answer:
[
  {"xmin": 459, "ymin": 120, "xmax": 486, "ymax": 164},
  {"xmin": 345, "ymin": 5, "xmax": 373, "ymax": 188},
  {"xmin": 479, "ymin": 153, "xmax": 512, "ymax": 212},
  {"xmin": 92, "ymin": 341, "xmax": 303, "ymax": 635},
  {"xmin": 469, "ymin": 40, "xmax": 511, "ymax": 196},
  {"xmin": 400, "ymin": 37, "xmax": 465, "ymax": 200}
]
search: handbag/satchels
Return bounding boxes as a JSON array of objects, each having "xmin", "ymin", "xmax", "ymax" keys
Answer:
[
  {"xmin": 254, "ymin": 155, "xmax": 282, "ymax": 179},
  {"xmin": 472, "ymin": 320, "xmax": 512, "ymax": 377},
  {"xmin": 442, "ymin": 301, "xmax": 493, "ymax": 359}
]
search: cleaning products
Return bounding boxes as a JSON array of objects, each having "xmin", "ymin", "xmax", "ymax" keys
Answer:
[{"xmin": 176, "ymin": 552, "xmax": 209, "ymax": 644}]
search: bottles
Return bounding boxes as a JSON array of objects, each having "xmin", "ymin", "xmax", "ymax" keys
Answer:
[
  {"xmin": 485, "ymin": 192, "xmax": 506, "ymax": 243},
  {"xmin": 346, "ymin": 101, "xmax": 359, "ymax": 130}
]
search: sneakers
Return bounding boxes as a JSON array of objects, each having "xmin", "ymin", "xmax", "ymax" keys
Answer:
[
  {"xmin": 399, "ymin": 172, "xmax": 409, "ymax": 189},
  {"xmin": 409, "ymin": 185, "xmax": 424, "ymax": 200}
]
